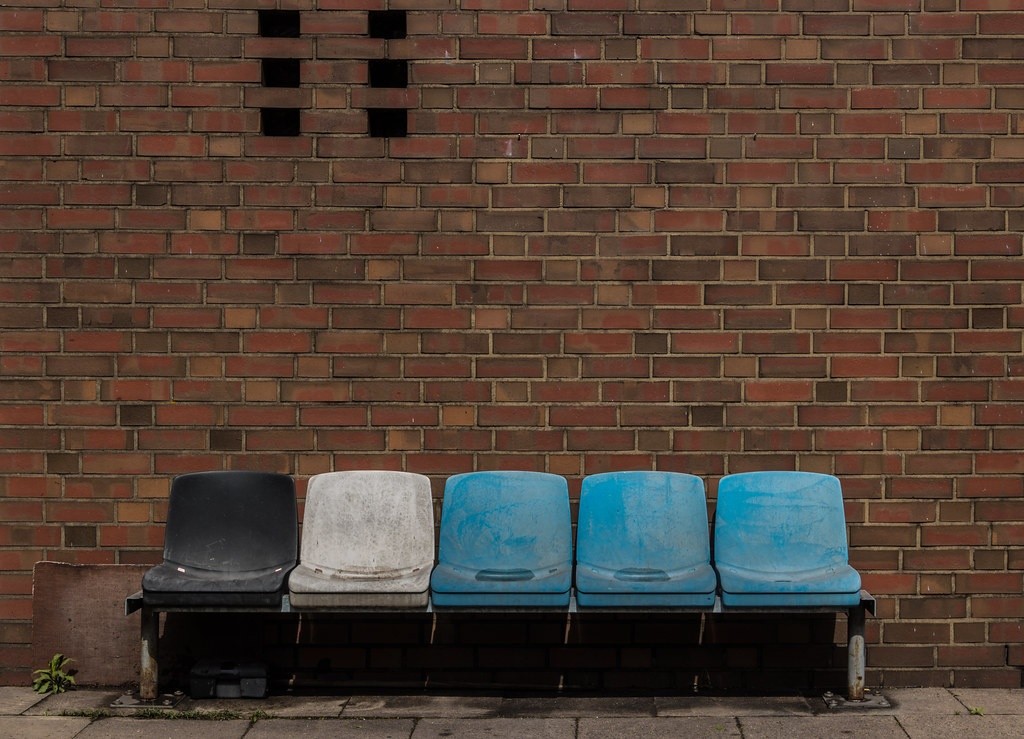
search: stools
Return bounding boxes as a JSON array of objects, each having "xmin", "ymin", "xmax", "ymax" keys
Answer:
[
  {"xmin": 286, "ymin": 468, "xmax": 437, "ymax": 607},
  {"xmin": 713, "ymin": 470, "xmax": 863, "ymax": 606},
  {"xmin": 575, "ymin": 469, "xmax": 718, "ymax": 606},
  {"xmin": 141, "ymin": 468, "xmax": 298, "ymax": 606},
  {"xmin": 429, "ymin": 469, "xmax": 573, "ymax": 607}
]
